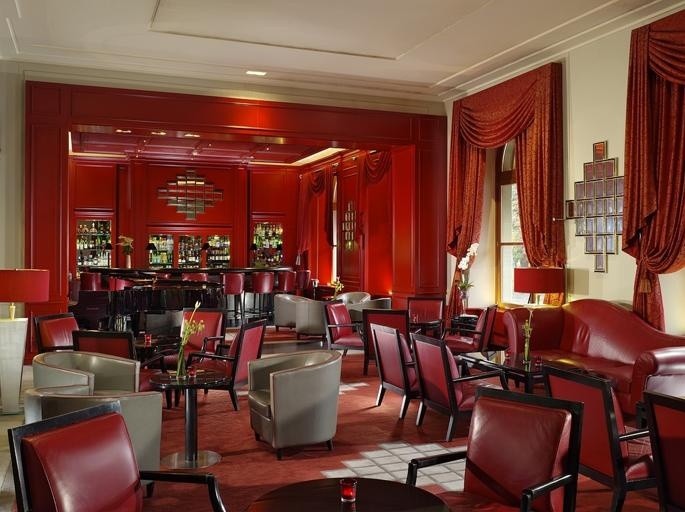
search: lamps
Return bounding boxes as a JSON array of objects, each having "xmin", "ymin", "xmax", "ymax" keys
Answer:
[{"xmin": 0, "ymin": 267, "xmax": 51, "ymax": 415}]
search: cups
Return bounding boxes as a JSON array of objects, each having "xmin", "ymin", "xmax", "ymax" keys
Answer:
[
  {"xmin": 339, "ymin": 478, "xmax": 357, "ymax": 504},
  {"xmin": 504, "ymin": 349, "xmax": 513, "ymax": 358},
  {"xmin": 535, "ymin": 354, "xmax": 543, "ymax": 366},
  {"xmin": 341, "ymin": 503, "xmax": 356, "ymax": 512},
  {"xmin": 188, "ymin": 366, "xmax": 196, "ymax": 378},
  {"xmin": 411, "ymin": 313, "xmax": 418, "ymax": 323},
  {"xmin": 144, "ymin": 333, "xmax": 151, "ymax": 343}
]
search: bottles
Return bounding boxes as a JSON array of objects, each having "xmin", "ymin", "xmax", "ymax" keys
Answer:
[
  {"xmin": 205, "ymin": 234, "xmax": 231, "ymax": 269},
  {"xmin": 77, "ymin": 220, "xmax": 112, "ymax": 277},
  {"xmin": 177, "ymin": 234, "xmax": 201, "ymax": 269},
  {"xmin": 147, "ymin": 233, "xmax": 174, "ymax": 270},
  {"xmin": 253, "ymin": 222, "xmax": 284, "ymax": 267}
]
